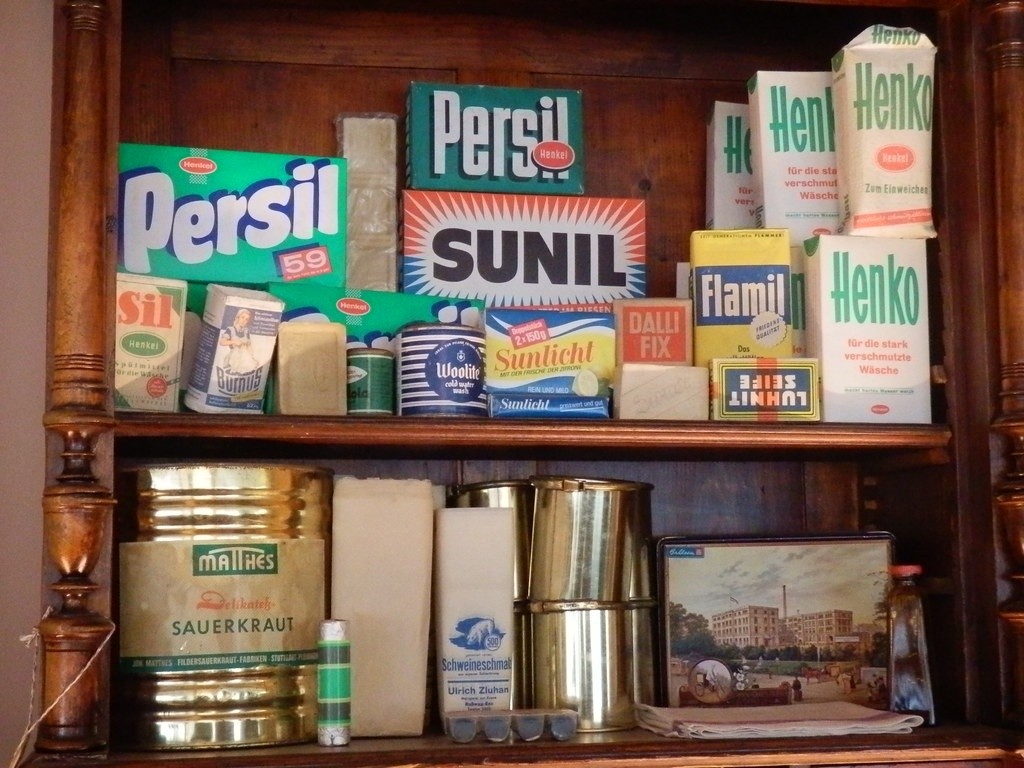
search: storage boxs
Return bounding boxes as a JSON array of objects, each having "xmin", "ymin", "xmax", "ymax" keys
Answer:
[{"xmin": 101, "ymin": 1, "xmax": 978, "ymax": 768}]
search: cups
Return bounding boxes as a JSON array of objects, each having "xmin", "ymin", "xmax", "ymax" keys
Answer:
[{"xmin": 439, "ymin": 473, "xmax": 657, "ymax": 733}]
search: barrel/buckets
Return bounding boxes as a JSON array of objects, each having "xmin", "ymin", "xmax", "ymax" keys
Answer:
[{"xmin": 115, "ymin": 460, "xmax": 335, "ymax": 750}]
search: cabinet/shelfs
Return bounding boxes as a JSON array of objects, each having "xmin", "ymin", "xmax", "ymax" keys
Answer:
[{"xmin": 22, "ymin": 0, "xmax": 1024, "ymax": 768}]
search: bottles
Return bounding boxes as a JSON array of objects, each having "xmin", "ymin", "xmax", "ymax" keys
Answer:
[{"xmin": 884, "ymin": 565, "xmax": 936, "ymax": 726}]
